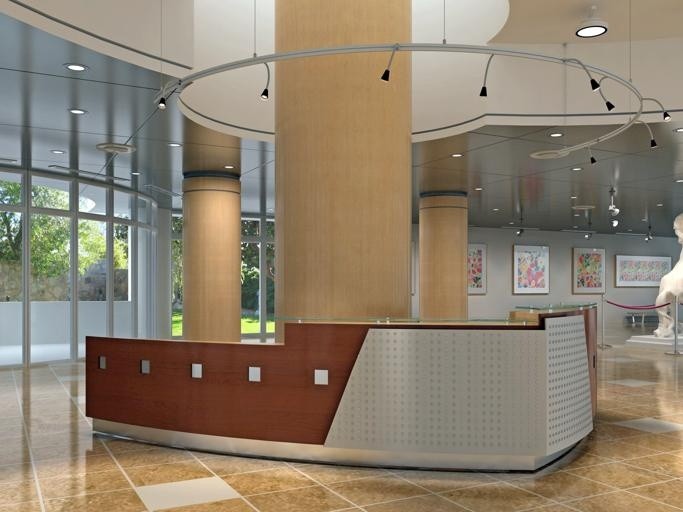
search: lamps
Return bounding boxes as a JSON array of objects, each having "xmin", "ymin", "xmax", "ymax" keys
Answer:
[
  {"xmin": 596, "ymin": 76, "xmax": 616, "ymax": 112},
  {"xmin": 587, "ymin": 145, "xmax": 596, "ymax": 163},
  {"xmin": 380, "ymin": 49, "xmax": 395, "ymax": 81},
  {"xmin": 641, "ymin": 97, "xmax": 670, "ymax": 121},
  {"xmin": 565, "ymin": 58, "xmax": 601, "ymax": 92},
  {"xmin": 479, "ymin": 54, "xmax": 494, "ymax": 97},
  {"xmin": 640, "ymin": 119, "xmax": 658, "ymax": 149},
  {"xmin": 160, "ymin": 89, "xmax": 167, "ymax": 110},
  {"xmin": 261, "ymin": 62, "xmax": 271, "ymax": 99}
]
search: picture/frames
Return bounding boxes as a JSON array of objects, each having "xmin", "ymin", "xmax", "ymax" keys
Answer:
[
  {"xmin": 615, "ymin": 254, "xmax": 672, "ymax": 288},
  {"xmin": 572, "ymin": 247, "xmax": 606, "ymax": 294},
  {"xmin": 513, "ymin": 244, "xmax": 550, "ymax": 295},
  {"xmin": 467, "ymin": 242, "xmax": 487, "ymax": 295}
]
